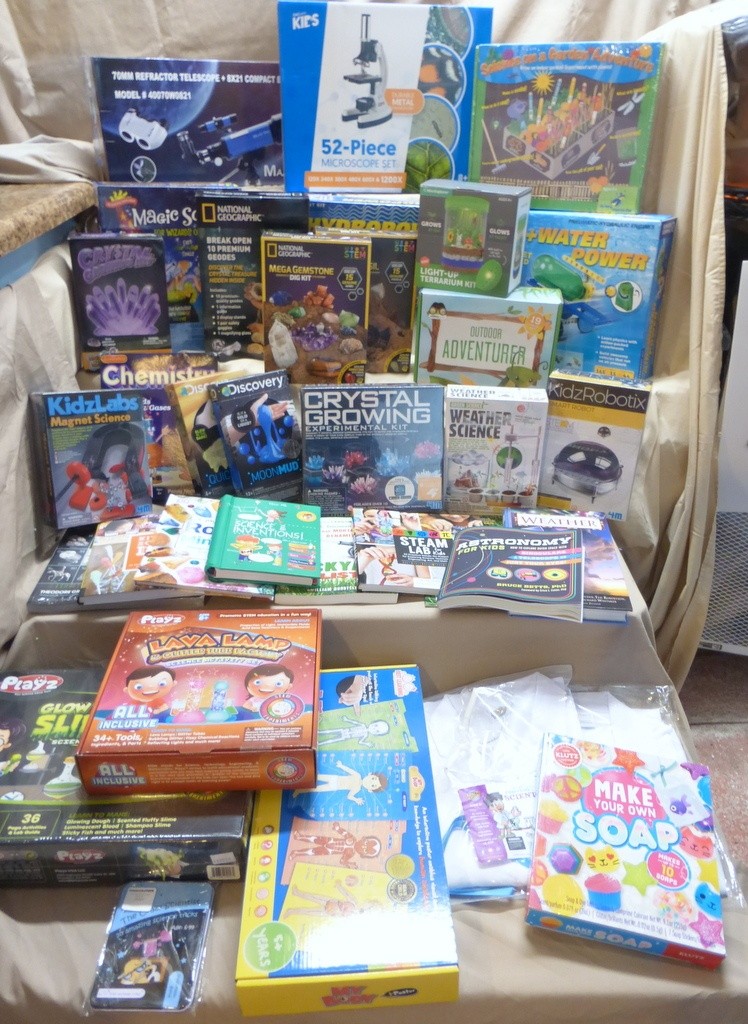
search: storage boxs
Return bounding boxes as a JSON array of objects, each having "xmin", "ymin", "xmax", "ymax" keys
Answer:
[
  {"xmin": 0, "ymin": 609, "xmax": 459, "ymax": 1018},
  {"xmin": 30, "ymin": 0, "xmax": 680, "ymax": 533},
  {"xmin": 525, "ymin": 733, "xmax": 726, "ymax": 971}
]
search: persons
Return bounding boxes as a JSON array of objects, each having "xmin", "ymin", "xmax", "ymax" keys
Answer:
[
  {"xmin": 351, "ymin": 508, "xmax": 484, "ymax": 587},
  {"xmin": 221, "ymin": 393, "xmax": 291, "ymax": 446},
  {"xmin": 98, "ymin": 518, "xmax": 137, "ymax": 537}
]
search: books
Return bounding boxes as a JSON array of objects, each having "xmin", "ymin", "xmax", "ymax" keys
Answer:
[{"xmin": 26, "ymin": 494, "xmax": 634, "ymax": 625}]
